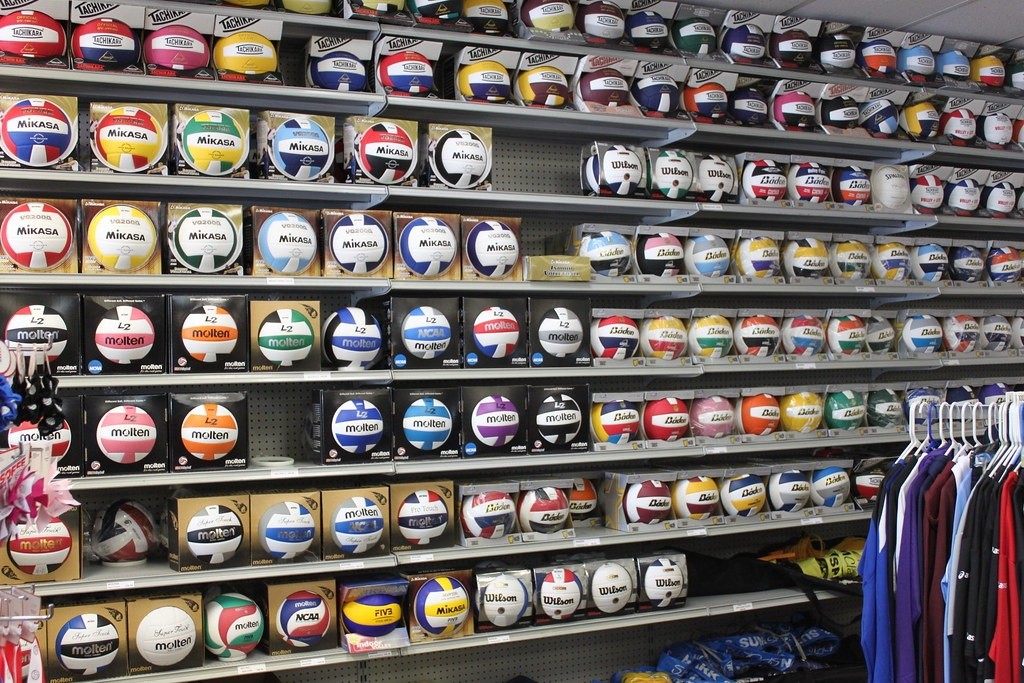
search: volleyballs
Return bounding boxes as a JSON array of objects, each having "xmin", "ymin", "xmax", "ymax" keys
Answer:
[
  {"xmin": 95, "ymin": 107, "xmax": 162, "ymax": 168},
  {"xmin": 4, "ymin": 304, "xmax": 67, "ymax": 363},
  {"xmin": 0, "ymin": 10, "xmax": 67, "ymax": 68},
  {"xmin": 57, "ymin": 612, "xmax": 120, "ymax": 675},
  {"xmin": 10, "ymin": 411, "xmax": 69, "ymax": 462},
  {"xmin": 4, "ymin": 98, "xmax": 71, "ymax": 165},
  {"xmin": 143, "ymin": 0, "xmax": 1024, "ymax": 666},
  {"xmin": 137, "ymin": 608, "xmax": 197, "ymax": 664},
  {"xmin": 70, "ymin": 17, "xmax": 142, "ymax": 68},
  {"xmin": 88, "ymin": 205, "xmax": 156, "ymax": 271},
  {"xmin": 94, "ymin": 305, "xmax": 155, "ymax": 364},
  {"xmin": 9, "ymin": 516, "xmax": 71, "ymax": 575},
  {"xmin": 0, "ymin": 201, "xmax": 74, "ymax": 273},
  {"xmin": 96, "ymin": 406, "xmax": 156, "ymax": 463},
  {"xmin": 94, "ymin": 500, "xmax": 157, "ymax": 565}
]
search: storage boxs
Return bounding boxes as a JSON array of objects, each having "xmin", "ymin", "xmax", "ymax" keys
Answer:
[{"xmin": 0, "ymin": 0, "xmax": 1023, "ymax": 683}]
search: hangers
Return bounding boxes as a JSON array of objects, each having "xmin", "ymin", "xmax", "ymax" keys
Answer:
[{"xmin": 894, "ymin": 391, "xmax": 1024, "ymax": 483}]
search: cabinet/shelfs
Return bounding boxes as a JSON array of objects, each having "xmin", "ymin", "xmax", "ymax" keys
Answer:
[{"xmin": 0, "ymin": 0, "xmax": 1024, "ymax": 683}]
